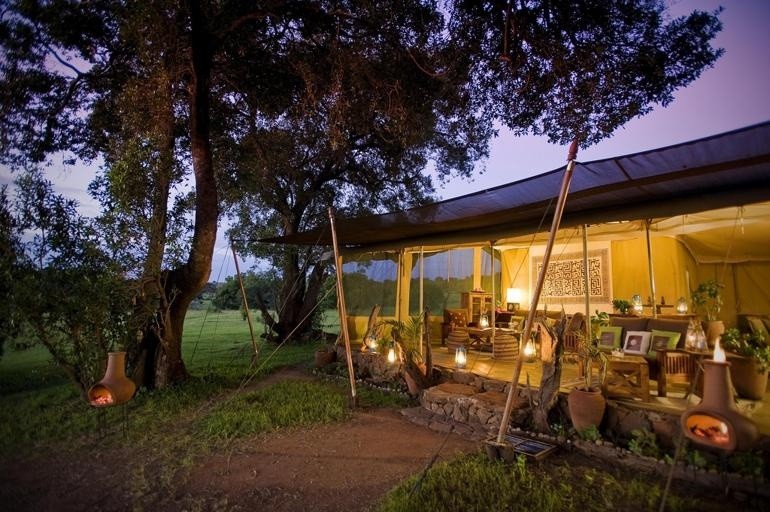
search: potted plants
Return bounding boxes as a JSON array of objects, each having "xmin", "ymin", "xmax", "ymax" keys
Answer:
[
  {"xmin": 311, "ymin": 272, "xmax": 344, "ymax": 367},
  {"xmin": 548, "ymin": 309, "xmax": 609, "ymax": 437},
  {"xmin": 690, "ymin": 277, "xmax": 770, "ymax": 399}
]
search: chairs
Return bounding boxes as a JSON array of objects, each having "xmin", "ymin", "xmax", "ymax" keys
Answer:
[{"xmin": 442, "ymin": 308, "xmax": 478, "ymax": 344}]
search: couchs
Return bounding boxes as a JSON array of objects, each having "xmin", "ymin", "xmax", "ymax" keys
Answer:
[
  {"xmin": 591, "ymin": 316, "xmax": 694, "ymax": 381},
  {"xmin": 495, "ymin": 310, "xmax": 563, "ymax": 340}
]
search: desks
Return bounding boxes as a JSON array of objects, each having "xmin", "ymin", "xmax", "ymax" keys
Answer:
[
  {"xmin": 467, "ymin": 327, "xmax": 514, "ymax": 346},
  {"xmin": 599, "ymin": 352, "xmax": 650, "ymax": 399}
]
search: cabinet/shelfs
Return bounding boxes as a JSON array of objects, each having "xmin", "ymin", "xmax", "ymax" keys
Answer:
[{"xmin": 462, "ymin": 293, "xmax": 492, "ymax": 324}]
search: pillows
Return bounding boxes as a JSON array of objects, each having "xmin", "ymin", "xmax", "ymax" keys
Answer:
[
  {"xmin": 507, "ymin": 315, "xmax": 524, "ymax": 331},
  {"xmin": 597, "ymin": 326, "xmax": 681, "ymax": 356}
]
